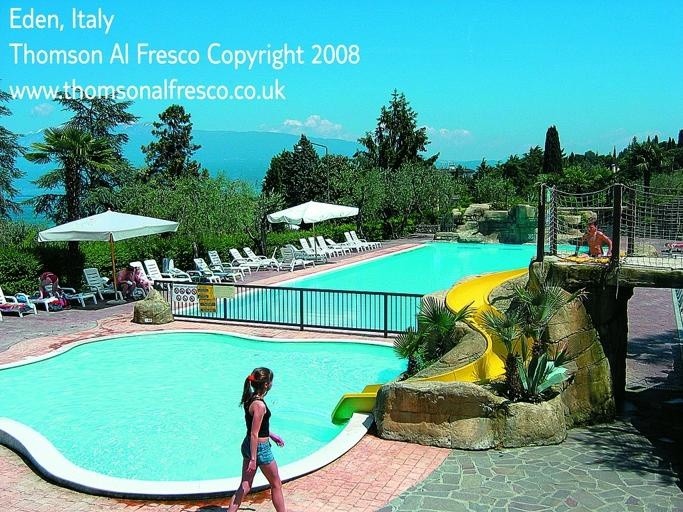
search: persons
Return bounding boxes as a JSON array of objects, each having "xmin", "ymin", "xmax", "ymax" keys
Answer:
[
  {"xmin": 226, "ymin": 366, "xmax": 287, "ymax": 512},
  {"xmin": 569, "ymin": 216, "xmax": 612, "ymax": 257},
  {"xmin": 116, "ymin": 265, "xmax": 136, "ymax": 301},
  {"xmin": 38, "ymin": 271, "xmax": 59, "ymax": 300},
  {"xmin": 135, "ymin": 268, "xmax": 152, "ymax": 292}
]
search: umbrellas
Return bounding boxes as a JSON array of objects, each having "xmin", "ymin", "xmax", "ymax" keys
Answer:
[
  {"xmin": 37, "ymin": 207, "xmax": 180, "ymax": 301},
  {"xmin": 266, "ymin": 199, "xmax": 360, "ymax": 262}
]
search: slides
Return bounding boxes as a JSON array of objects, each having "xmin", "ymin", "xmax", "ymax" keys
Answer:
[{"xmin": 331, "ymin": 250, "xmax": 628, "ymax": 426}]
